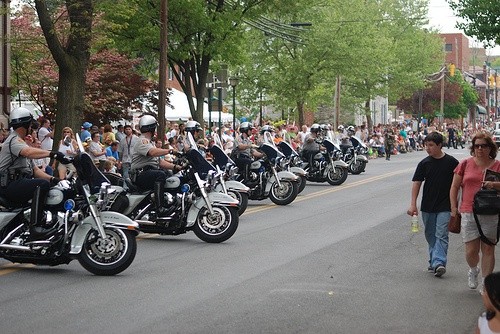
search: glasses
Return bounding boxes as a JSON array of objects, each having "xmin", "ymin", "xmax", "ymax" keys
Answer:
[{"xmin": 474, "ymin": 143, "xmax": 489, "ymax": 149}]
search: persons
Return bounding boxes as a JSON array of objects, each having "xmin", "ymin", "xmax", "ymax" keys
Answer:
[{"xmin": 0, "ymin": 107, "xmax": 500, "ymax": 334}]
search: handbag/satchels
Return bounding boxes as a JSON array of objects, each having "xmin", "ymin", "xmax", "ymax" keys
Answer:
[
  {"xmin": 448, "ymin": 213, "xmax": 460, "ymax": 233},
  {"xmin": 473, "ymin": 189, "xmax": 499, "ymax": 246}
]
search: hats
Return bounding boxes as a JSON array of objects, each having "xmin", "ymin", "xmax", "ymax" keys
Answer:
[{"xmin": 83, "ymin": 122, "xmax": 92, "ymax": 128}]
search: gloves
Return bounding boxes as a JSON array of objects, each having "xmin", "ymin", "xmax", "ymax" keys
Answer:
[
  {"xmin": 50, "ymin": 150, "xmax": 72, "ymax": 164},
  {"xmin": 173, "ymin": 150, "xmax": 184, "ymax": 158}
]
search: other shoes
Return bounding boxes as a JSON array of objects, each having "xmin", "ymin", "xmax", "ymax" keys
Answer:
[
  {"xmin": 428, "ymin": 258, "xmax": 434, "ymax": 273},
  {"xmin": 480, "ymin": 276, "xmax": 486, "ymax": 296},
  {"xmin": 467, "ymin": 266, "xmax": 479, "ymax": 289},
  {"xmin": 433, "ymin": 264, "xmax": 446, "ymax": 277}
]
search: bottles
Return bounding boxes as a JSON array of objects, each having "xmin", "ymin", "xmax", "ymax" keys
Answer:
[{"xmin": 411, "ymin": 211, "xmax": 419, "ymax": 233}]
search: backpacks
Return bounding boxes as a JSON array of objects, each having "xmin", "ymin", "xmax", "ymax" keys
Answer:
[{"xmin": 50, "ymin": 176, "xmax": 63, "ymax": 184}]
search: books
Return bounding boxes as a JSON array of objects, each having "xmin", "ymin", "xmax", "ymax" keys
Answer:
[{"xmin": 481, "ymin": 168, "xmax": 500, "ymax": 191}]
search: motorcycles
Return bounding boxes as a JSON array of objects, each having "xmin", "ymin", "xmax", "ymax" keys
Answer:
[
  {"xmin": 102, "ymin": 131, "xmax": 368, "ymax": 245},
  {"xmin": 0, "ymin": 132, "xmax": 140, "ymax": 276}
]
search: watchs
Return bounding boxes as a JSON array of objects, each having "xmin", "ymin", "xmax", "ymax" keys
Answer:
[{"xmin": 169, "ymin": 149, "xmax": 173, "ymax": 154}]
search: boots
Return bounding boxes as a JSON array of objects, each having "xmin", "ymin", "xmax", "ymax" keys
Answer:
[
  {"xmin": 241, "ymin": 162, "xmax": 256, "ymax": 184},
  {"xmin": 153, "ymin": 181, "xmax": 176, "ymax": 216},
  {"xmin": 29, "ymin": 185, "xmax": 58, "ymax": 240},
  {"xmin": 308, "ymin": 154, "xmax": 317, "ymax": 172}
]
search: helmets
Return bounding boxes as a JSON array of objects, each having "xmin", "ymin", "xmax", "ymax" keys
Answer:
[
  {"xmin": 185, "ymin": 120, "xmax": 200, "ymax": 131},
  {"xmin": 239, "ymin": 122, "xmax": 255, "ymax": 133},
  {"xmin": 338, "ymin": 125, "xmax": 344, "ymax": 131},
  {"xmin": 310, "ymin": 123, "xmax": 321, "ymax": 132},
  {"xmin": 139, "ymin": 114, "xmax": 160, "ymax": 132},
  {"xmin": 348, "ymin": 126, "xmax": 354, "ymax": 132},
  {"xmin": 259, "ymin": 125, "xmax": 275, "ymax": 135},
  {"xmin": 9, "ymin": 107, "xmax": 34, "ymax": 126}
]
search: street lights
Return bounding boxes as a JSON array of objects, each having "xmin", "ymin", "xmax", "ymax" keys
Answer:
[{"xmin": 229, "ymin": 74, "xmax": 240, "ymax": 139}]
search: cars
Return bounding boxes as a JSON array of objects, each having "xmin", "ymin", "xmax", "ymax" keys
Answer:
[{"xmin": 492, "ymin": 129, "xmax": 500, "ymax": 148}]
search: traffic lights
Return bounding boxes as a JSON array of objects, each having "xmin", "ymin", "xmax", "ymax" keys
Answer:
[{"xmin": 488, "ymin": 74, "xmax": 494, "ymax": 90}]
